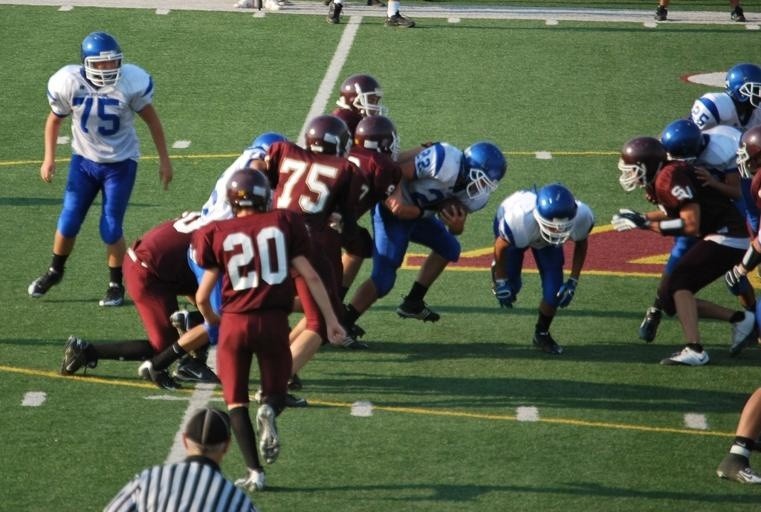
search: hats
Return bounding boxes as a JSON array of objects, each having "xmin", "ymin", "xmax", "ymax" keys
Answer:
[{"xmin": 182, "ymin": 405, "xmax": 235, "ymax": 446}]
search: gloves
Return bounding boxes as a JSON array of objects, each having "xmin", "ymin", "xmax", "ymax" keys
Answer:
[
  {"xmin": 610, "ymin": 206, "xmax": 652, "ymax": 233},
  {"xmin": 418, "ymin": 202, "xmax": 440, "ymax": 221},
  {"xmin": 555, "ymin": 276, "xmax": 579, "ymax": 309},
  {"xmin": 492, "ymin": 278, "xmax": 518, "ymax": 309}
]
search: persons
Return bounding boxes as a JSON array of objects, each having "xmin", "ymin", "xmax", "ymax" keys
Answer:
[
  {"xmin": 65, "ymin": 74, "xmax": 506, "ymax": 465},
  {"xmin": 612, "ymin": 65, "xmax": 761, "ymax": 484},
  {"xmin": 492, "ymin": 184, "xmax": 596, "ymax": 355},
  {"xmin": 654, "ymin": 0, "xmax": 746, "ymax": 22},
  {"xmin": 29, "ymin": 34, "xmax": 174, "ymax": 307},
  {"xmin": 103, "ymin": 406, "xmax": 260, "ymax": 512},
  {"xmin": 327, "ymin": 0, "xmax": 415, "ymax": 28}
]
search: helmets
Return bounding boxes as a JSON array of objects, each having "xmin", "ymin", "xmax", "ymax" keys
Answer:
[
  {"xmin": 722, "ymin": 62, "xmax": 761, "ymax": 109},
  {"xmin": 735, "ymin": 123, "xmax": 761, "ymax": 180},
  {"xmin": 225, "ymin": 167, "xmax": 271, "ymax": 211},
  {"xmin": 340, "ymin": 73, "xmax": 391, "ymax": 119},
  {"xmin": 303, "ymin": 113, "xmax": 353, "ymax": 158},
  {"xmin": 461, "ymin": 138, "xmax": 509, "ymax": 201},
  {"xmin": 80, "ymin": 31, "xmax": 125, "ymax": 89},
  {"xmin": 251, "ymin": 132, "xmax": 290, "ymax": 153},
  {"xmin": 532, "ymin": 183, "xmax": 579, "ymax": 247},
  {"xmin": 354, "ymin": 114, "xmax": 401, "ymax": 164},
  {"xmin": 659, "ymin": 117, "xmax": 706, "ymax": 162},
  {"xmin": 616, "ymin": 136, "xmax": 668, "ymax": 194}
]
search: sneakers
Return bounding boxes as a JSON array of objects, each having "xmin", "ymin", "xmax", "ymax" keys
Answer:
[
  {"xmin": 60, "ymin": 332, "xmax": 93, "ymax": 377},
  {"xmin": 716, "ymin": 449, "xmax": 761, "ymax": 487},
  {"xmin": 253, "ymin": 402, "xmax": 283, "ymax": 466},
  {"xmin": 335, "ymin": 307, "xmax": 371, "ymax": 350},
  {"xmin": 26, "ymin": 263, "xmax": 66, "ymax": 299},
  {"xmin": 637, "ymin": 302, "xmax": 761, "ymax": 368},
  {"xmin": 253, "ymin": 370, "xmax": 309, "ymax": 409},
  {"xmin": 324, "ymin": 0, "xmax": 416, "ymax": 29},
  {"xmin": 97, "ymin": 278, "xmax": 126, "ymax": 308},
  {"xmin": 136, "ymin": 309, "xmax": 222, "ymax": 394},
  {"xmin": 234, "ymin": 0, "xmax": 284, "ymax": 11},
  {"xmin": 393, "ymin": 296, "xmax": 442, "ymax": 324},
  {"xmin": 653, "ymin": 3, "xmax": 748, "ymax": 22},
  {"xmin": 229, "ymin": 468, "xmax": 266, "ymax": 495},
  {"xmin": 531, "ymin": 325, "xmax": 566, "ymax": 358}
]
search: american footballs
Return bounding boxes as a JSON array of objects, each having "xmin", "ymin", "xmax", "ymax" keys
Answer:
[{"xmin": 439, "ymin": 198, "xmax": 463, "ymax": 218}]
